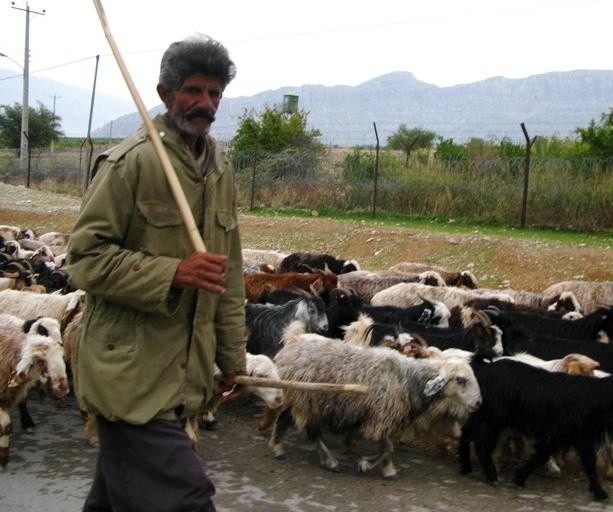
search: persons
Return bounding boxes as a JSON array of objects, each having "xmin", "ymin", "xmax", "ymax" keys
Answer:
[{"xmin": 59, "ymin": 34, "xmax": 250, "ymax": 512}]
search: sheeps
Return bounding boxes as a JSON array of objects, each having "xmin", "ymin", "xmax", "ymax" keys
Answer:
[
  {"xmin": 239, "ymin": 248, "xmax": 613, "ymax": 501},
  {"xmin": 62, "ymin": 310, "xmax": 284, "ymax": 451},
  {"xmin": 0, "ymin": 224, "xmax": 92, "ymax": 471}
]
